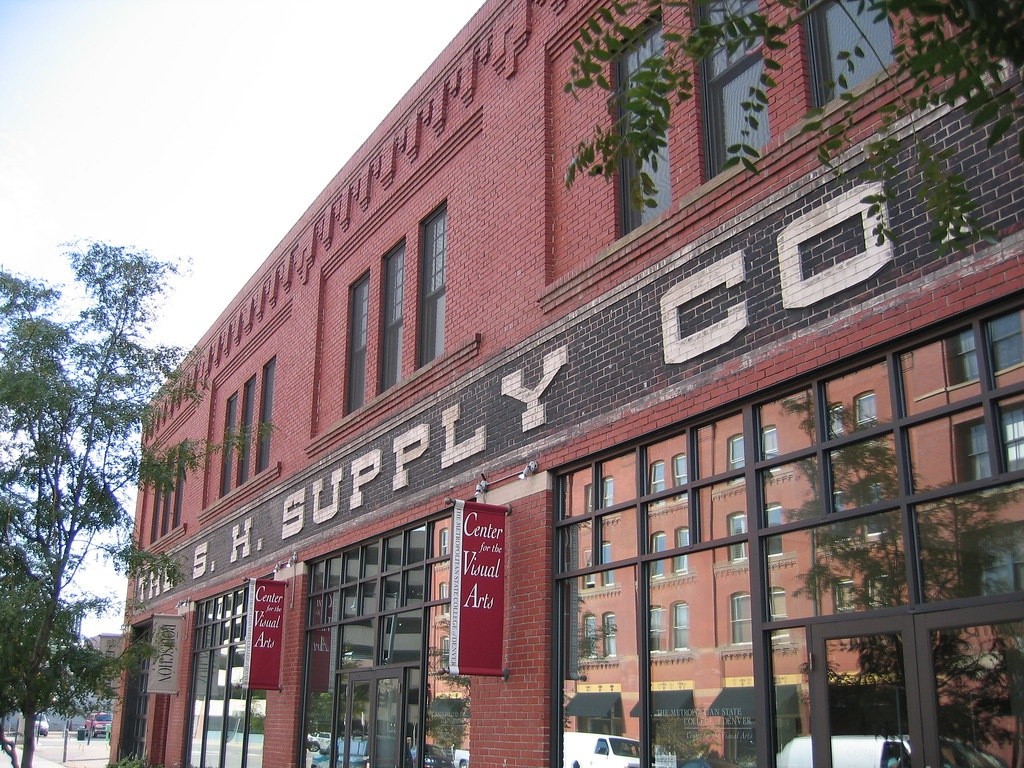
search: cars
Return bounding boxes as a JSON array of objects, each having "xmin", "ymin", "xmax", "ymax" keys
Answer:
[
  {"xmin": 34, "ymin": 713, "xmax": 50, "ymax": 737},
  {"xmin": 306, "ymin": 731, "xmax": 332, "ymax": 752},
  {"xmin": 310, "ymin": 735, "xmax": 369, "ymax": 768},
  {"xmin": 410, "ymin": 744, "xmax": 454, "ymax": 768},
  {"xmin": 454, "ymin": 749, "xmax": 468, "ymax": 768}
]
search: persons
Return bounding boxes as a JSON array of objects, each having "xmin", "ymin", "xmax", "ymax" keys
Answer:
[{"xmin": 887, "ymin": 746, "xmax": 901, "ymax": 768}]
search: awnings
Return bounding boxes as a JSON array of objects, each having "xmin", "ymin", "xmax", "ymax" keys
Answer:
[
  {"xmin": 629, "ymin": 689, "xmax": 696, "ymax": 718},
  {"xmin": 706, "ymin": 685, "xmax": 801, "ymax": 717},
  {"xmin": 564, "ymin": 691, "xmax": 623, "ymax": 718}
]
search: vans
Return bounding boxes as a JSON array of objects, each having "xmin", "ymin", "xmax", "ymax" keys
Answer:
[
  {"xmin": 562, "ymin": 731, "xmax": 642, "ymax": 768},
  {"xmin": 772, "ymin": 732, "xmax": 1006, "ymax": 768},
  {"xmin": 84, "ymin": 712, "xmax": 113, "ymax": 737}
]
